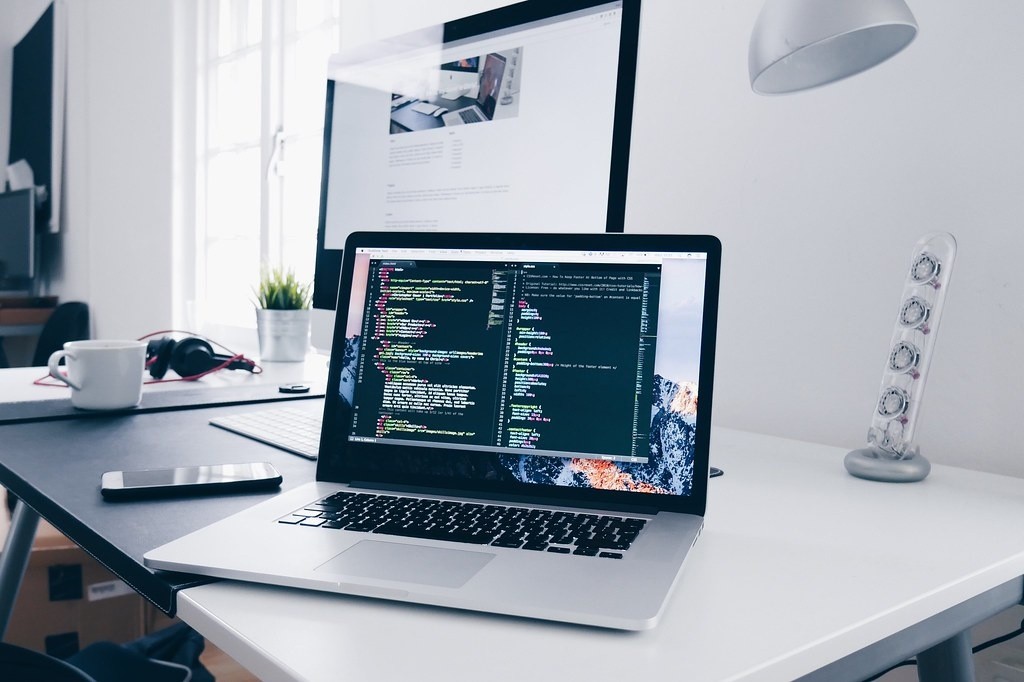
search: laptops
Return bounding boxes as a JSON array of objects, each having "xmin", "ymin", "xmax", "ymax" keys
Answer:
[{"xmin": 144, "ymin": 230, "xmax": 721, "ymax": 632}]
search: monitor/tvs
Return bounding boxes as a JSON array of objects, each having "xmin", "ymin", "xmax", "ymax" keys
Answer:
[
  {"xmin": 310, "ymin": 0, "xmax": 641, "ymax": 353},
  {"xmin": 0, "ymin": 187, "xmax": 35, "ymax": 291}
]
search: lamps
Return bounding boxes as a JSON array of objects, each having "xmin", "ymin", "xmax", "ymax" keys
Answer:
[
  {"xmin": 748, "ymin": 0, "xmax": 918, "ymax": 95},
  {"xmin": 844, "ymin": 231, "xmax": 957, "ymax": 483},
  {"xmin": 500, "ymin": 47, "xmax": 522, "ymax": 105}
]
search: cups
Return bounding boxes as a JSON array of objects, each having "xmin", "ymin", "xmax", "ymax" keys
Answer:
[{"xmin": 48, "ymin": 339, "xmax": 148, "ymax": 409}]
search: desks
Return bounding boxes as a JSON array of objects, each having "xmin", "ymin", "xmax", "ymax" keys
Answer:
[
  {"xmin": 0, "ymin": 352, "xmax": 1024, "ymax": 682},
  {"xmin": 0, "ymin": 308, "xmax": 55, "ymax": 337},
  {"xmin": 389, "ymin": 95, "xmax": 491, "ymax": 135}
]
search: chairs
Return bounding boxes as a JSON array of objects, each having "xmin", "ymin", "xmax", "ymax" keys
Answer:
[{"xmin": 31, "ymin": 302, "xmax": 90, "ymax": 367}]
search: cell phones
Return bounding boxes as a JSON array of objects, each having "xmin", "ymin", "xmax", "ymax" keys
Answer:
[{"xmin": 102, "ymin": 461, "xmax": 283, "ymax": 499}]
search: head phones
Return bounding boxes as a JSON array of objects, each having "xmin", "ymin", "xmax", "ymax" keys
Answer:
[{"xmin": 144, "ymin": 337, "xmax": 255, "ymax": 381}]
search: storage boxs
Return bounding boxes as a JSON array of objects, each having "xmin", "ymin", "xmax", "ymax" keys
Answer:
[{"xmin": 0, "ymin": 538, "xmax": 144, "ymax": 659}]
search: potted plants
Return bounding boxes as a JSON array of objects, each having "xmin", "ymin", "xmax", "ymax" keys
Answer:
[{"xmin": 251, "ymin": 265, "xmax": 315, "ymax": 362}]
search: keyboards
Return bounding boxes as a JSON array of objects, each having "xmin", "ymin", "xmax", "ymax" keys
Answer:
[{"xmin": 207, "ymin": 406, "xmax": 321, "ymax": 460}]
